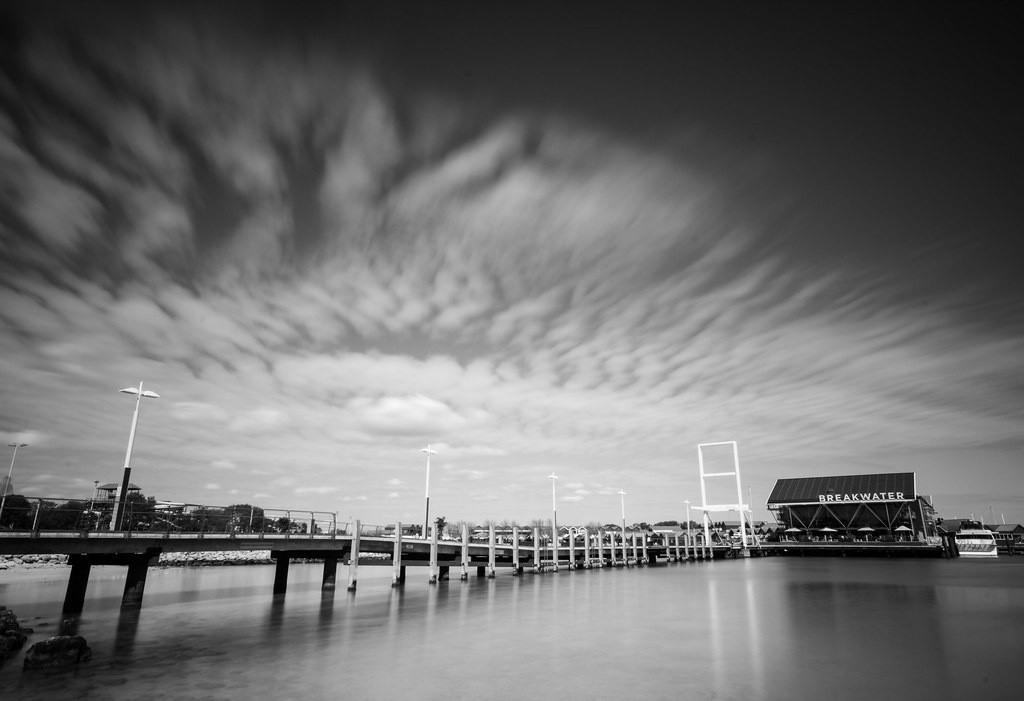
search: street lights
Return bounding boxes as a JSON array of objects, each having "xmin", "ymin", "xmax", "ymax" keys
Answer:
[
  {"xmin": 684, "ymin": 500, "xmax": 691, "ymax": 545},
  {"xmin": 618, "ymin": 488, "xmax": 626, "ymax": 547},
  {"xmin": 0, "ymin": 442, "xmax": 29, "ymax": 522},
  {"xmin": 421, "ymin": 444, "xmax": 436, "ymax": 539},
  {"xmin": 547, "ymin": 471, "xmax": 557, "ymax": 549},
  {"xmin": 110, "ymin": 381, "xmax": 160, "ymax": 532},
  {"xmin": 747, "ymin": 486, "xmax": 754, "ymax": 544}
]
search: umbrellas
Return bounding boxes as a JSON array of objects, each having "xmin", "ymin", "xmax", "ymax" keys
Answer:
[
  {"xmin": 818, "ymin": 527, "xmax": 837, "ymax": 532},
  {"xmin": 857, "ymin": 527, "xmax": 874, "ymax": 531},
  {"xmin": 895, "ymin": 525, "xmax": 912, "ymax": 531},
  {"xmin": 784, "ymin": 528, "xmax": 802, "ymax": 533}
]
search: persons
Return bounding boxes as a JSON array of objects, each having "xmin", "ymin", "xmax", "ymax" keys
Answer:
[{"xmin": 935, "ymin": 517, "xmax": 942, "ymax": 525}]
